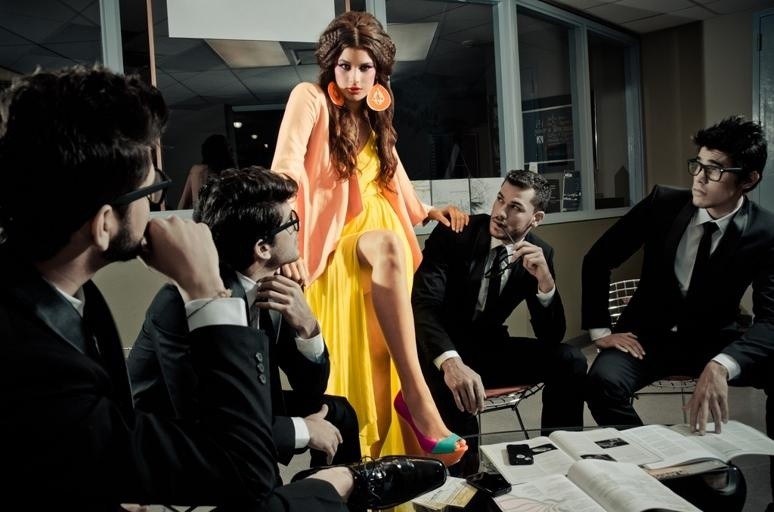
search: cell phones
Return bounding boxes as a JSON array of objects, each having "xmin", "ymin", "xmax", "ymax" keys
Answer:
[
  {"xmin": 507, "ymin": 443, "xmax": 535, "ymax": 466},
  {"xmin": 466, "ymin": 471, "xmax": 512, "ymax": 498}
]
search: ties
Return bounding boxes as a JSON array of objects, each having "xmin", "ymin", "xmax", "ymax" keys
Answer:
[
  {"xmin": 687, "ymin": 222, "xmax": 718, "ymax": 303},
  {"xmin": 484, "ymin": 247, "xmax": 506, "ymax": 313},
  {"xmin": 258, "ymin": 297, "xmax": 282, "ymax": 391}
]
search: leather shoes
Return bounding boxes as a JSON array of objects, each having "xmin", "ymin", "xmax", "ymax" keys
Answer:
[{"xmin": 291, "ymin": 456, "xmax": 446, "ymax": 510}]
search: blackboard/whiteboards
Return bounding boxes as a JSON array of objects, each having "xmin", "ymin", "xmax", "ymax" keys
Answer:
[{"xmin": 522, "ymin": 104, "xmax": 575, "ymax": 165}]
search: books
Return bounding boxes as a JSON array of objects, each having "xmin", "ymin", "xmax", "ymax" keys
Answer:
[
  {"xmin": 477, "ymin": 427, "xmax": 667, "ymax": 486},
  {"xmin": 429, "ymin": 178, "xmax": 471, "ymax": 216},
  {"xmin": 470, "ymin": 177, "xmax": 504, "ymax": 216},
  {"xmin": 491, "ymin": 458, "xmax": 706, "ymax": 512},
  {"xmin": 409, "ymin": 180, "xmax": 434, "ymax": 227},
  {"xmin": 637, "ymin": 460, "xmax": 730, "ymax": 480},
  {"xmin": 618, "ymin": 417, "xmax": 773, "ymax": 470}
]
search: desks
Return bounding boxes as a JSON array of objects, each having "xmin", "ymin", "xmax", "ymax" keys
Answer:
[{"xmin": 370, "ymin": 425, "xmax": 774, "ymax": 511}]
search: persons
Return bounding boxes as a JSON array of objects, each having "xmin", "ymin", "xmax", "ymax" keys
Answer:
[
  {"xmin": 579, "ymin": 115, "xmax": 774, "ymax": 511},
  {"xmin": 411, "ymin": 167, "xmax": 587, "ymax": 473},
  {"xmin": 1, "ymin": 60, "xmax": 447, "ymax": 512},
  {"xmin": 124, "ymin": 164, "xmax": 359, "ymax": 481},
  {"xmin": 269, "ymin": 8, "xmax": 470, "ymax": 464},
  {"xmin": 172, "ymin": 134, "xmax": 235, "ymax": 213}
]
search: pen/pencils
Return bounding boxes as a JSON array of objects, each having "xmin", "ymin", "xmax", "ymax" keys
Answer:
[{"xmin": 500, "ymin": 225, "xmax": 519, "ymax": 250}]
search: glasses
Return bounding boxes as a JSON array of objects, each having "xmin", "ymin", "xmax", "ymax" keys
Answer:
[
  {"xmin": 112, "ymin": 168, "xmax": 171, "ymax": 207},
  {"xmin": 264, "ymin": 209, "xmax": 300, "ymax": 240},
  {"xmin": 686, "ymin": 157, "xmax": 749, "ymax": 182}
]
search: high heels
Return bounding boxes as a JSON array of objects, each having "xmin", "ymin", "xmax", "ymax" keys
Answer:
[{"xmin": 394, "ymin": 389, "xmax": 469, "ymax": 467}]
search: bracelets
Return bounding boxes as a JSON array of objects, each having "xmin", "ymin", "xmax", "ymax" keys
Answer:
[{"xmin": 185, "ymin": 289, "xmax": 231, "ymax": 321}]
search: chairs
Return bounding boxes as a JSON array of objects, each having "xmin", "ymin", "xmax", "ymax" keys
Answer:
[{"xmin": 477, "ymin": 279, "xmax": 716, "ymax": 438}]
